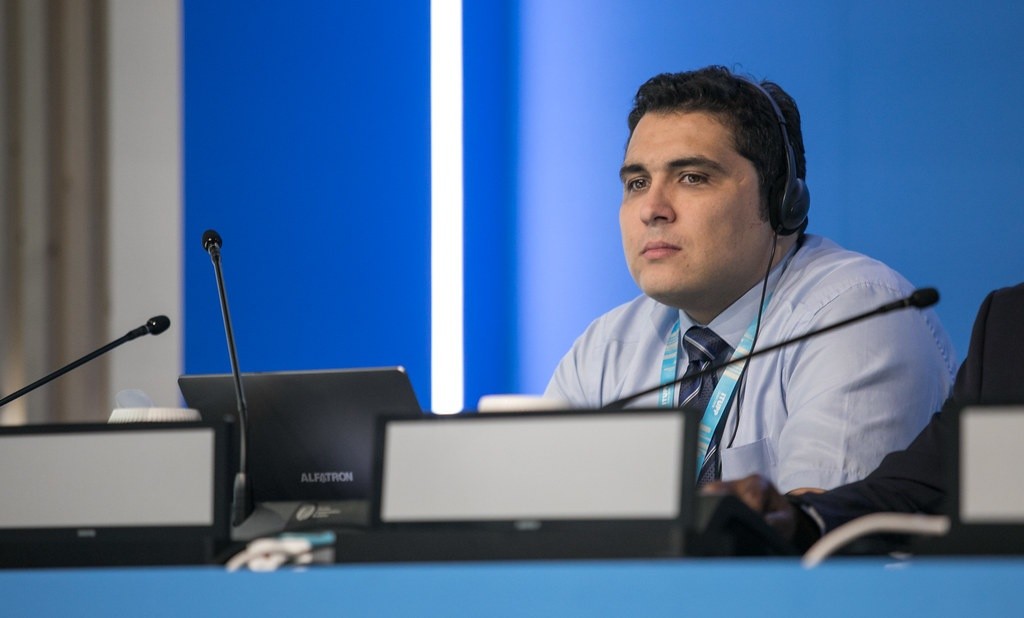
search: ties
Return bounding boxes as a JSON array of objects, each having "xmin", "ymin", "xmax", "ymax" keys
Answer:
[{"xmin": 678, "ymin": 325, "xmax": 730, "ymax": 491}]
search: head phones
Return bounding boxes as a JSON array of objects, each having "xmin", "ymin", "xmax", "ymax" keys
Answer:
[{"xmin": 736, "ymin": 75, "xmax": 811, "ymax": 237}]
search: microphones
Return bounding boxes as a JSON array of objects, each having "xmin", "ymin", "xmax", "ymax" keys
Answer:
[
  {"xmin": 0, "ymin": 315, "xmax": 170, "ymax": 406},
  {"xmin": 203, "ymin": 230, "xmax": 247, "ymax": 528},
  {"xmin": 602, "ymin": 288, "xmax": 941, "ymax": 411}
]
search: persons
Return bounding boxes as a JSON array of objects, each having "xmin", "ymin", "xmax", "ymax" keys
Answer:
[
  {"xmin": 541, "ymin": 65, "xmax": 944, "ymax": 494},
  {"xmin": 699, "ymin": 282, "xmax": 1023, "ymax": 558}
]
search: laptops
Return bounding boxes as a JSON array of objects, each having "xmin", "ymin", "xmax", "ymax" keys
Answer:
[{"xmin": 178, "ymin": 367, "xmax": 424, "ymax": 502}]
